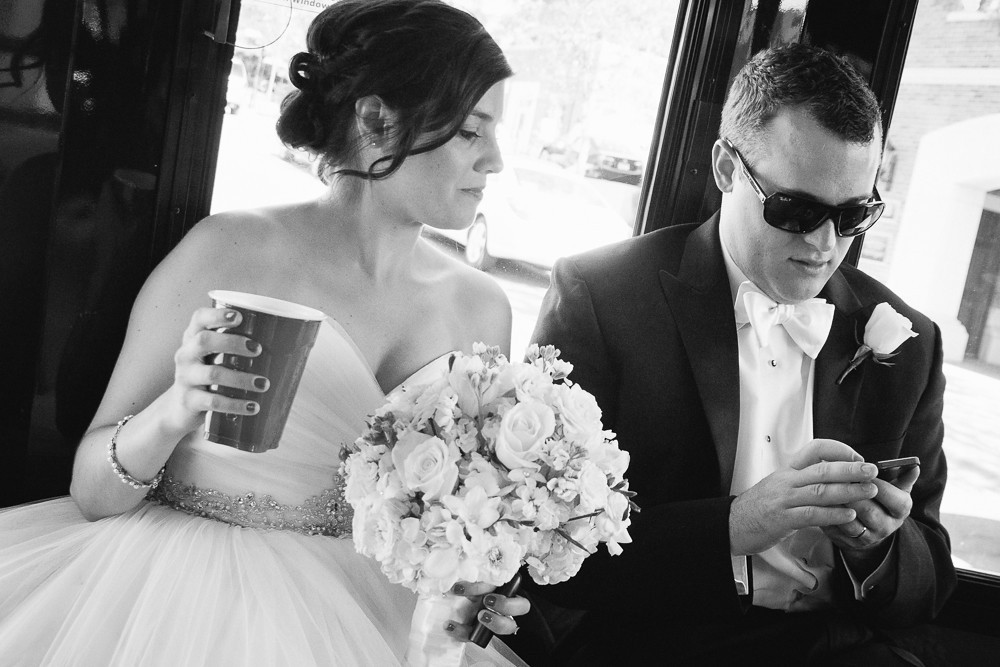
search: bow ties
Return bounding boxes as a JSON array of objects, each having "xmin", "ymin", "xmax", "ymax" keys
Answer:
[{"xmin": 732, "ymin": 279, "xmax": 836, "ymax": 359}]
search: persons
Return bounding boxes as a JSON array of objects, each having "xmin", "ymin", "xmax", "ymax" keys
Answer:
[
  {"xmin": 495, "ymin": 36, "xmax": 959, "ymax": 667},
  {"xmin": 67, "ymin": 0, "xmax": 532, "ymax": 666}
]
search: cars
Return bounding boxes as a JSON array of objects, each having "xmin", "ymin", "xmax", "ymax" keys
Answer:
[{"xmin": 315, "ymin": 133, "xmax": 624, "ymax": 280}]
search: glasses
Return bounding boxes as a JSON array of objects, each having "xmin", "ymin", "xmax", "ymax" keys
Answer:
[{"xmin": 723, "ymin": 138, "xmax": 886, "ymax": 239}]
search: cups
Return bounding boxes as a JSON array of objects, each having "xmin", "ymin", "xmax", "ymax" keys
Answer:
[{"xmin": 201, "ymin": 289, "xmax": 326, "ymax": 454}]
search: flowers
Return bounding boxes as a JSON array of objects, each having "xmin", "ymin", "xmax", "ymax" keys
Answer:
[
  {"xmin": 834, "ymin": 301, "xmax": 920, "ymax": 387},
  {"xmin": 341, "ymin": 337, "xmax": 641, "ymax": 667}
]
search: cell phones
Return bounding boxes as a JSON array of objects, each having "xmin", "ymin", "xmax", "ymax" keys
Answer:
[{"xmin": 874, "ymin": 457, "xmax": 921, "ymax": 485}]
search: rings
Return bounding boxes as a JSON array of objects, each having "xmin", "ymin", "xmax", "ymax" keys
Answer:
[{"xmin": 849, "ymin": 526, "xmax": 867, "ymax": 539}]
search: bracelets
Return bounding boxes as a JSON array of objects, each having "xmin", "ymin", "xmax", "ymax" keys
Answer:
[{"xmin": 107, "ymin": 415, "xmax": 167, "ymax": 490}]
